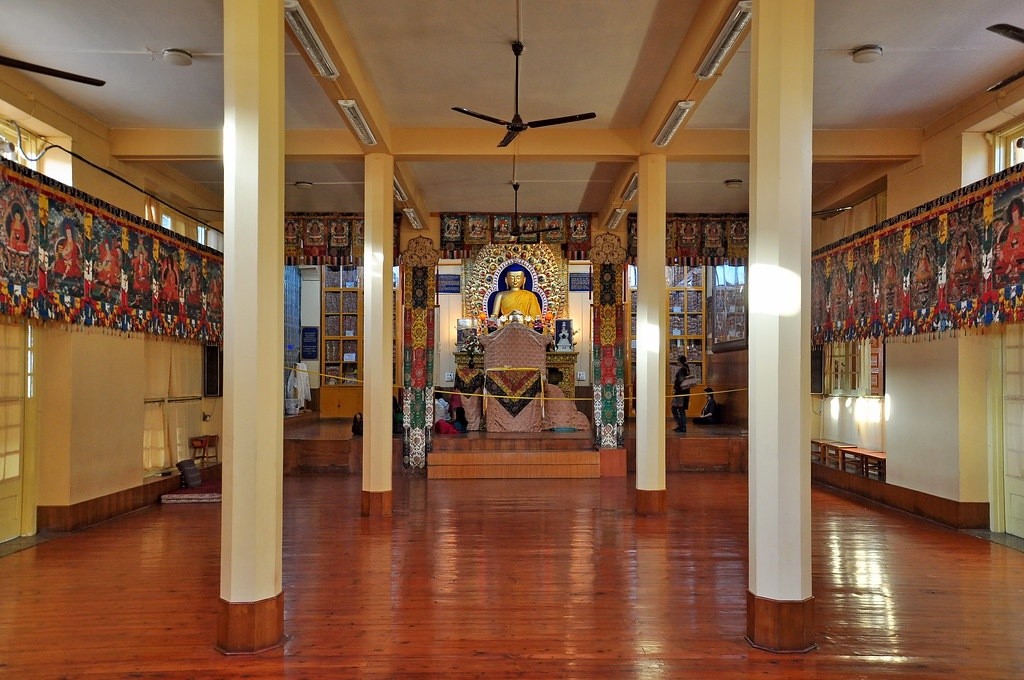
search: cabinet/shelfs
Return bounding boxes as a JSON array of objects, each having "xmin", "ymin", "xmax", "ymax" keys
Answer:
[
  {"xmin": 625, "ymin": 264, "xmax": 708, "ymax": 419},
  {"xmin": 319, "ymin": 265, "xmax": 402, "ymax": 421}
]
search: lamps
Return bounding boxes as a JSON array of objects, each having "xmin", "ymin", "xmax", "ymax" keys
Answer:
[
  {"xmin": 403, "ymin": 208, "xmax": 423, "ymax": 229},
  {"xmin": 725, "ymin": 179, "xmax": 742, "ymax": 189},
  {"xmin": 607, "ymin": 209, "xmax": 627, "ymax": 230},
  {"xmin": 337, "ymin": 99, "xmax": 378, "ymax": 146},
  {"xmin": 694, "ymin": 0, "xmax": 752, "ymax": 82},
  {"xmin": 393, "ymin": 175, "xmax": 408, "ymax": 202},
  {"xmin": 623, "ymin": 172, "xmax": 638, "ymax": 203},
  {"xmin": 852, "ymin": 44, "xmax": 883, "ymax": 63},
  {"xmin": 653, "ymin": 100, "xmax": 695, "ymax": 147},
  {"xmin": 295, "ymin": 182, "xmax": 313, "ymax": 189},
  {"xmin": 163, "ymin": 48, "xmax": 192, "ymax": 66},
  {"xmin": 0, "ymin": 55, "xmax": 106, "ymax": 87},
  {"xmin": 284, "ymin": 0, "xmax": 340, "ymax": 81}
]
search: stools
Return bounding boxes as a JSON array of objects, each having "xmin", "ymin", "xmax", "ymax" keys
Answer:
[{"xmin": 811, "ymin": 439, "xmax": 886, "ymax": 482}]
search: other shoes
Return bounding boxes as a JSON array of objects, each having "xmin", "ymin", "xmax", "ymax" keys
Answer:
[{"xmin": 672, "ymin": 426, "xmax": 686, "ymax": 433}]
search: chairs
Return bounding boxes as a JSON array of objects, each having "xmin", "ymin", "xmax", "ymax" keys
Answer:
[{"xmin": 205, "ymin": 434, "xmax": 220, "ymax": 465}]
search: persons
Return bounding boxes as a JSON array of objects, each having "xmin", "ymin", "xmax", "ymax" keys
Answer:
[
  {"xmin": 393, "ymin": 394, "xmax": 402, "ymax": 433},
  {"xmin": 434, "ymin": 387, "xmax": 461, "ymax": 422},
  {"xmin": 671, "ymin": 355, "xmax": 692, "ymax": 434},
  {"xmin": 492, "ymin": 262, "xmax": 542, "ymax": 323},
  {"xmin": 692, "ymin": 387, "xmax": 718, "ymax": 424}
]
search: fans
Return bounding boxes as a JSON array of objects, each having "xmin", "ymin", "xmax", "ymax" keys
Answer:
[
  {"xmin": 985, "ymin": 23, "xmax": 1024, "ymax": 92},
  {"xmin": 485, "ymin": 182, "xmax": 560, "ymax": 236},
  {"xmin": 451, "ymin": 42, "xmax": 597, "ymax": 147}
]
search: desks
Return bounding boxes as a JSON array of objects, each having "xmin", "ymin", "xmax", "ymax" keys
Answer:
[{"xmin": 188, "ymin": 436, "xmax": 219, "ymax": 466}]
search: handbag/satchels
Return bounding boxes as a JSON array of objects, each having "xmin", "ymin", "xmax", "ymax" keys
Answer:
[
  {"xmin": 680, "ymin": 367, "xmax": 697, "ymax": 390},
  {"xmin": 352, "ymin": 413, "xmax": 363, "ymax": 436}
]
